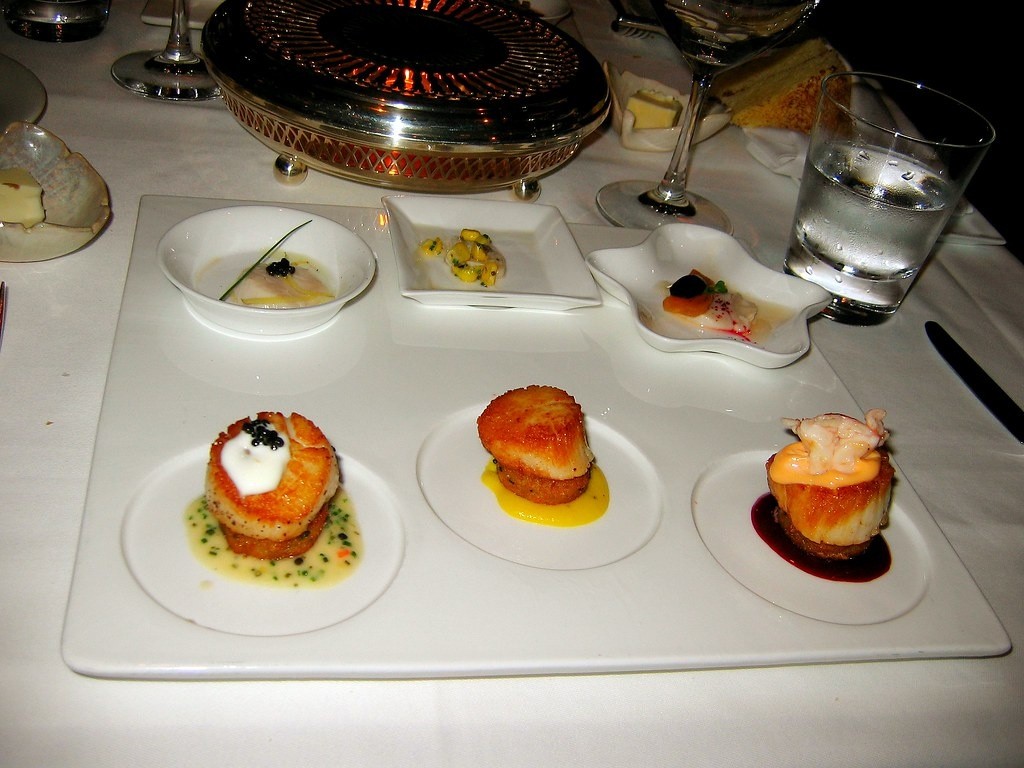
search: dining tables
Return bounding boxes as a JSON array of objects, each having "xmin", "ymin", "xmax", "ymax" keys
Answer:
[{"xmin": 0, "ymin": 0, "xmax": 1024, "ymax": 768}]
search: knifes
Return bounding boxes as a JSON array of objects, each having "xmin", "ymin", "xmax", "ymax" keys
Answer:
[{"xmin": 924, "ymin": 321, "xmax": 1024, "ymax": 443}]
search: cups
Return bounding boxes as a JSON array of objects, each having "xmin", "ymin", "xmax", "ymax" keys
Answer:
[
  {"xmin": 5, "ymin": 0, "xmax": 111, "ymax": 43},
  {"xmin": 781, "ymin": 71, "xmax": 995, "ymax": 328}
]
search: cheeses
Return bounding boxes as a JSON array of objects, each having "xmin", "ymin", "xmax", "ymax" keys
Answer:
[
  {"xmin": 0, "ymin": 167, "xmax": 46, "ymax": 228},
  {"xmin": 626, "ymin": 88, "xmax": 682, "ymax": 129}
]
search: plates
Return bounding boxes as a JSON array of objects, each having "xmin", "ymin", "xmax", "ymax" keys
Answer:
[
  {"xmin": 120, "ymin": 442, "xmax": 405, "ymax": 638},
  {"xmin": 0, "ymin": 122, "xmax": 113, "ymax": 263},
  {"xmin": 585, "ymin": 223, "xmax": 831, "ymax": 369},
  {"xmin": 691, "ymin": 448, "xmax": 933, "ymax": 625},
  {"xmin": 415, "ymin": 396, "xmax": 662, "ymax": 570},
  {"xmin": 0, "ymin": 53, "xmax": 47, "ymax": 135},
  {"xmin": 382, "ymin": 195, "xmax": 604, "ymax": 313},
  {"xmin": 600, "ymin": 61, "xmax": 734, "ymax": 149}
]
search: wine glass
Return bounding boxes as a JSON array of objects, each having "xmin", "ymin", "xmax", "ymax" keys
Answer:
[
  {"xmin": 596, "ymin": 0, "xmax": 820, "ymax": 236},
  {"xmin": 109, "ymin": 0, "xmax": 222, "ymax": 102}
]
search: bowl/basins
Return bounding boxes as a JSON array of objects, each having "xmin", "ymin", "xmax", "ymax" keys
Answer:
[
  {"xmin": 153, "ymin": 205, "xmax": 376, "ymax": 338},
  {"xmin": 523, "ymin": 0, "xmax": 572, "ymax": 25}
]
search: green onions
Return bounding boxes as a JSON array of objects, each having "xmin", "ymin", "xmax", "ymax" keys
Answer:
[{"xmin": 185, "ymin": 494, "xmax": 357, "ymax": 586}]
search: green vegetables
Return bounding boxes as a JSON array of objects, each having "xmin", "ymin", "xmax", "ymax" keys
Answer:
[{"xmin": 429, "ymin": 241, "xmax": 487, "ymax": 288}]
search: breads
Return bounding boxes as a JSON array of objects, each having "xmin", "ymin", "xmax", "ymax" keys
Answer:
[{"xmin": 710, "ymin": 38, "xmax": 852, "ymax": 138}]
